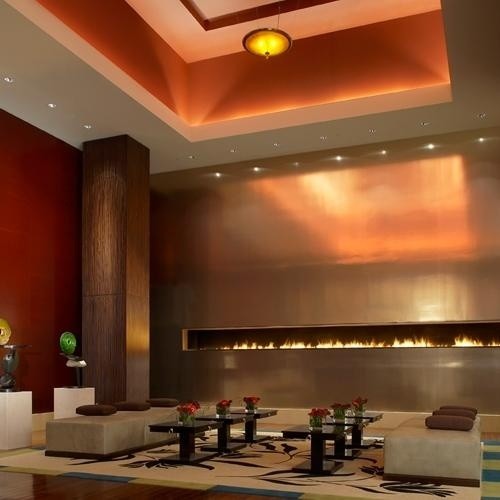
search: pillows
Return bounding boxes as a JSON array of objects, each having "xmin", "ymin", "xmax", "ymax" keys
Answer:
[
  {"xmin": 425, "ymin": 415, "xmax": 473, "ymax": 431},
  {"xmin": 115, "ymin": 400, "xmax": 150, "ymax": 411},
  {"xmin": 146, "ymin": 398, "xmax": 178, "ymax": 407},
  {"xmin": 439, "ymin": 405, "xmax": 478, "ymax": 417},
  {"xmin": 76, "ymin": 405, "xmax": 117, "ymax": 416},
  {"xmin": 432, "ymin": 409, "xmax": 476, "ymax": 421}
]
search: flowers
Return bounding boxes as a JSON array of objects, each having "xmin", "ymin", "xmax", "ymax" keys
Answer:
[
  {"xmin": 351, "ymin": 397, "xmax": 368, "ymax": 415},
  {"xmin": 243, "ymin": 397, "xmax": 260, "ymax": 415},
  {"xmin": 177, "ymin": 400, "xmax": 201, "ymax": 425},
  {"xmin": 331, "ymin": 403, "xmax": 351, "ymax": 420},
  {"xmin": 308, "ymin": 408, "xmax": 331, "ymax": 430}
]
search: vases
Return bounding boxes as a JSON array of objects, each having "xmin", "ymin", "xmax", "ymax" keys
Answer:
[{"xmin": 216, "ymin": 399, "xmax": 232, "ymax": 417}]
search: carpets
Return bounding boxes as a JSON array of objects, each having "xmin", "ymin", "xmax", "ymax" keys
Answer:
[{"xmin": 0, "ymin": 427, "xmax": 500, "ymax": 500}]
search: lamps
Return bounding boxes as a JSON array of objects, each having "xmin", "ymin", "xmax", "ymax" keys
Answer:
[{"xmin": 242, "ymin": 0, "xmax": 292, "ymax": 59}]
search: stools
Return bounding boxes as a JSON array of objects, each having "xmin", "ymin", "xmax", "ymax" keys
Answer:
[
  {"xmin": 383, "ymin": 414, "xmax": 481, "ymax": 487},
  {"xmin": 44, "ymin": 405, "xmax": 205, "ymax": 460}
]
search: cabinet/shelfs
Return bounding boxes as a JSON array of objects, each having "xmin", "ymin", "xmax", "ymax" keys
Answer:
[
  {"xmin": 0, "ymin": 390, "xmax": 32, "ymax": 451},
  {"xmin": 54, "ymin": 387, "xmax": 95, "ymax": 420}
]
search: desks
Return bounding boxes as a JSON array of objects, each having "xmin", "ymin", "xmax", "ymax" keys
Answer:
[
  {"xmin": 230, "ymin": 410, "xmax": 277, "ymax": 443},
  {"xmin": 281, "ymin": 425, "xmax": 353, "ymax": 475},
  {"xmin": 345, "ymin": 413, "xmax": 382, "ymax": 449},
  {"xmin": 195, "ymin": 415, "xmax": 254, "ymax": 453},
  {"xmin": 323, "ymin": 417, "xmax": 370, "ymax": 460},
  {"xmin": 149, "ymin": 421, "xmax": 218, "ymax": 465}
]
